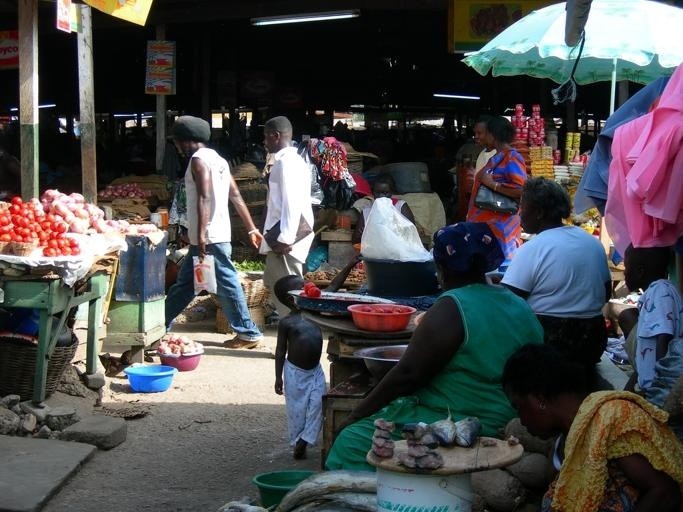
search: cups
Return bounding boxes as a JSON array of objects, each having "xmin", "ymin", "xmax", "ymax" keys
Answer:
[{"xmin": 151, "ymin": 214, "xmax": 162, "ymax": 227}]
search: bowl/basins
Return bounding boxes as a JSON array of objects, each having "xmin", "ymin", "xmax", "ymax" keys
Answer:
[
  {"xmin": 351, "ymin": 344, "xmax": 409, "ymax": 377},
  {"xmin": 346, "ymin": 303, "xmax": 417, "ymax": 332},
  {"xmin": 382, "ymin": 162, "xmax": 431, "ymax": 194},
  {"xmin": 251, "ymin": 470, "xmax": 322, "ymax": 508},
  {"xmin": 122, "ymin": 364, "xmax": 179, "ymax": 393},
  {"xmin": 159, "ymin": 352, "xmax": 202, "ymax": 371},
  {"xmin": 358, "ymin": 255, "xmax": 443, "ymax": 298},
  {"xmin": 609, "ymin": 299, "xmax": 640, "ymax": 317}
]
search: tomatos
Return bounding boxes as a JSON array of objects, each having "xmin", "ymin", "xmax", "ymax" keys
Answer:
[{"xmin": 0, "ymin": 196, "xmax": 81, "ymax": 257}]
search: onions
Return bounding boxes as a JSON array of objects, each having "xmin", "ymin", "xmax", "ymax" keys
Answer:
[{"xmin": 98, "ymin": 183, "xmax": 146, "ymax": 199}]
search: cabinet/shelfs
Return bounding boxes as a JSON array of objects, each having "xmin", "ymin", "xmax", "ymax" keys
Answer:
[{"xmin": 99, "ymin": 230, "xmax": 170, "ymax": 366}]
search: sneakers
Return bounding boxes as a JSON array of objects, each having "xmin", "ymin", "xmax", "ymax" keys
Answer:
[{"xmin": 222, "ymin": 333, "xmax": 260, "ymax": 350}]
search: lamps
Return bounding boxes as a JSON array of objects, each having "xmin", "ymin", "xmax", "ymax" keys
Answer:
[{"xmin": 249, "ymin": 9, "xmax": 362, "ymax": 26}]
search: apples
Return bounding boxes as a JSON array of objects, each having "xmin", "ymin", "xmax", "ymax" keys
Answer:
[{"xmin": 304, "ymin": 281, "xmax": 320, "ymax": 297}]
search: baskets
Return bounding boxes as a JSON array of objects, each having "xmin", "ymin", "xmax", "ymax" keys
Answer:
[
  {"xmin": 206, "ymin": 268, "xmax": 268, "ymax": 308},
  {"xmin": 2, "ymin": 314, "xmax": 77, "ymax": 403},
  {"xmin": 214, "ymin": 309, "xmax": 267, "ymax": 332}
]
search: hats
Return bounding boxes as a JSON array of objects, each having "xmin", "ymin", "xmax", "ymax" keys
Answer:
[{"xmin": 166, "ymin": 115, "xmax": 211, "ymax": 143}]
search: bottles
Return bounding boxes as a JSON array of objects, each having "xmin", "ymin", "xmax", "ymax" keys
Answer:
[
  {"xmin": 158, "ymin": 206, "xmax": 169, "ymax": 228},
  {"xmin": 577, "ymin": 113, "xmax": 582, "ymax": 127},
  {"xmin": 586, "ymin": 114, "xmax": 595, "ymax": 127}
]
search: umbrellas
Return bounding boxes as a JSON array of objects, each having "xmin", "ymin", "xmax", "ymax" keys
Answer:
[{"xmin": 459, "ymin": 1, "xmax": 682, "ymax": 115}]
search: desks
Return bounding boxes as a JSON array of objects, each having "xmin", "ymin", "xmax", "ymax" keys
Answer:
[{"xmin": 0, "ymin": 265, "xmax": 109, "ymax": 405}]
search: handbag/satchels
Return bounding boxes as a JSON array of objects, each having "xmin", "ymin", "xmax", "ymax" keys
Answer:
[{"xmin": 474, "ymin": 149, "xmax": 520, "ymax": 213}]
